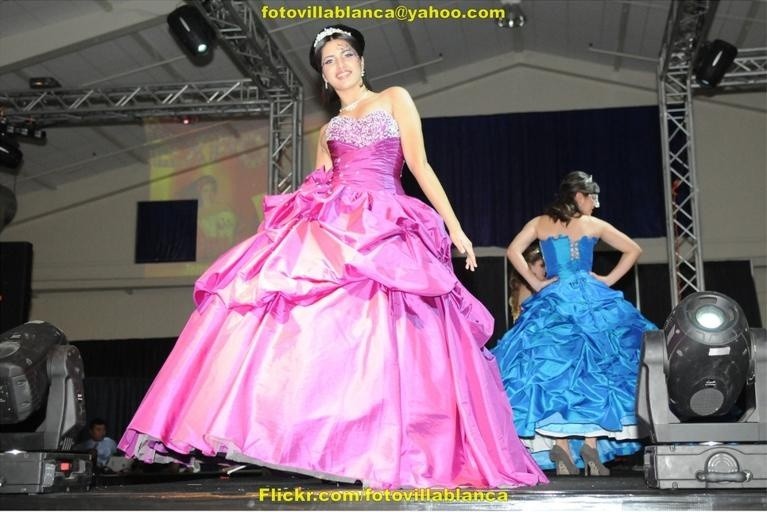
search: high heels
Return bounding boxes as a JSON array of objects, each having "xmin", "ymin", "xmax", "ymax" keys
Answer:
[
  {"xmin": 550, "ymin": 445, "xmax": 580, "ymax": 475},
  {"xmin": 579, "ymin": 444, "xmax": 610, "ymax": 476}
]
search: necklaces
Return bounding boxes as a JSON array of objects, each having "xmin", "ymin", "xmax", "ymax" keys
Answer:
[{"xmin": 339, "ymin": 91, "xmax": 374, "ymax": 113}]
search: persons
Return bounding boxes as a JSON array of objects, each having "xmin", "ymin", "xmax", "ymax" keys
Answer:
[
  {"xmin": 489, "ymin": 168, "xmax": 656, "ymax": 478},
  {"xmin": 506, "ymin": 246, "xmax": 548, "ymax": 325},
  {"xmin": 73, "ymin": 419, "xmax": 120, "ymax": 470},
  {"xmin": 117, "ymin": 24, "xmax": 551, "ymax": 489}
]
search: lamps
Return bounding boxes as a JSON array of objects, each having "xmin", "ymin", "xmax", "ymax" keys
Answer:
[
  {"xmin": 684, "ymin": 33, "xmax": 739, "ymax": 91},
  {"xmin": 0, "ymin": 318, "xmax": 101, "ymax": 497},
  {"xmin": 627, "ymin": 288, "xmax": 767, "ymax": 493},
  {"xmin": 164, "ymin": 2, "xmax": 218, "ymax": 60},
  {"xmin": 0, "ymin": 116, "xmax": 25, "ymax": 172}
]
